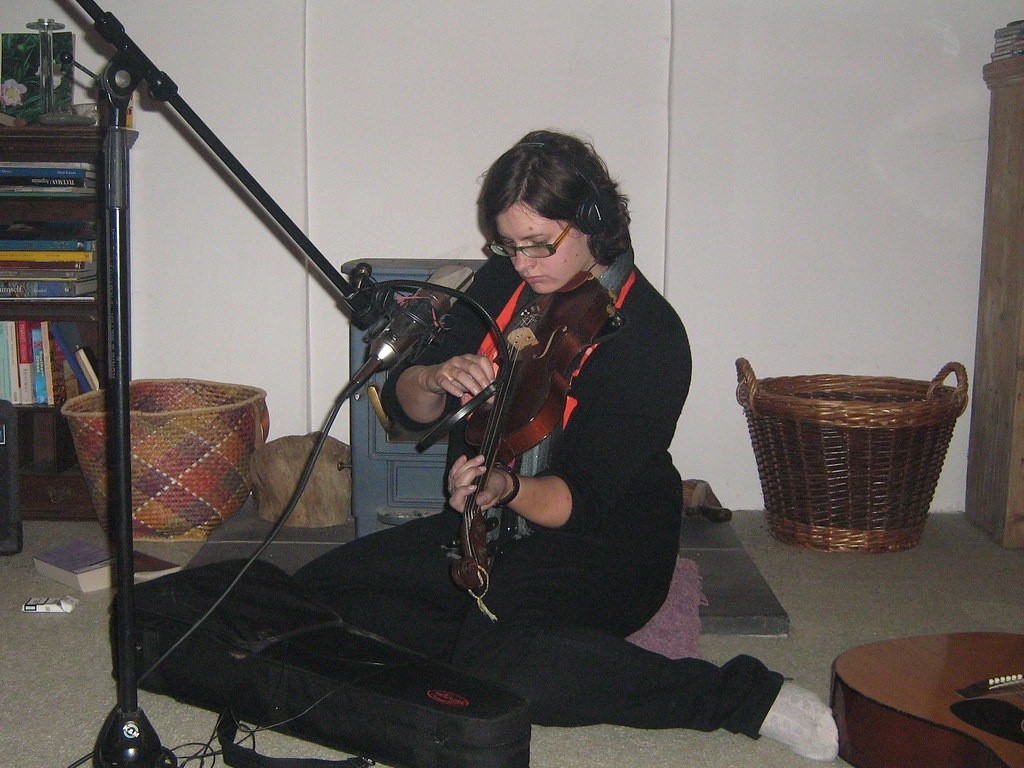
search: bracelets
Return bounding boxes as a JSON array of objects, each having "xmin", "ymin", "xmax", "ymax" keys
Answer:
[{"xmin": 492, "ymin": 470, "xmax": 520, "ymax": 509}]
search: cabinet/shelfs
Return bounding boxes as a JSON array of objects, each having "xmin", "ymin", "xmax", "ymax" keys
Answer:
[
  {"xmin": 341, "ymin": 257, "xmax": 557, "ymax": 537},
  {"xmin": 0, "ymin": 120, "xmax": 140, "ymax": 524}
]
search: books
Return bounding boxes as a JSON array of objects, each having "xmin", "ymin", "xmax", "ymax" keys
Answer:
[
  {"xmin": 133, "ymin": 551, "xmax": 182, "ymax": 580},
  {"xmin": 0, "ymin": 239, "xmax": 98, "ymax": 302},
  {"xmin": 0, "ymin": 161, "xmax": 102, "ymax": 197},
  {"xmin": 33, "ymin": 538, "xmax": 118, "ymax": 593},
  {"xmin": 0, "ymin": 320, "xmax": 101, "ymax": 405}
]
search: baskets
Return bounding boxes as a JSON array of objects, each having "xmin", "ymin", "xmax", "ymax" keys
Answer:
[
  {"xmin": 735, "ymin": 356, "xmax": 969, "ymax": 555},
  {"xmin": 61, "ymin": 377, "xmax": 269, "ymax": 542}
]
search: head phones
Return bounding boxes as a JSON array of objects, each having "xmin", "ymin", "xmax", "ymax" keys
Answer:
[{"xmin": 512, "ymin": 142, "xmax": 610, "ymax": 235}]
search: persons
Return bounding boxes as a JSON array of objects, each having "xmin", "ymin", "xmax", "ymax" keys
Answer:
[{"xmin": 290, "ymin": 130, "xmax": 838, "ymax": 762}]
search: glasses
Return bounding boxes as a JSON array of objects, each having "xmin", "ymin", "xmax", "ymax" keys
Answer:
[{"xmin": 488, "ymin": 223, "xmax": 575, "ymax": 258}]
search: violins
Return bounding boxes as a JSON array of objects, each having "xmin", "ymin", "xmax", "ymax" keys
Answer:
[{"xmin": 449, "ymin": 270, "xmax": 612, "ymax": 590}]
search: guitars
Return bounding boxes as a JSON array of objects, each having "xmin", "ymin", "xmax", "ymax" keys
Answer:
[{"xmin": 828, "ymin": 631, "xmax": 1024, "ymax": 768}]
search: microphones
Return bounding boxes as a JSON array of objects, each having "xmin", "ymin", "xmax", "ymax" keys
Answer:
[{"xmin": 341, "ymin": 262, "xmax": 474, "ymax": 397}]
